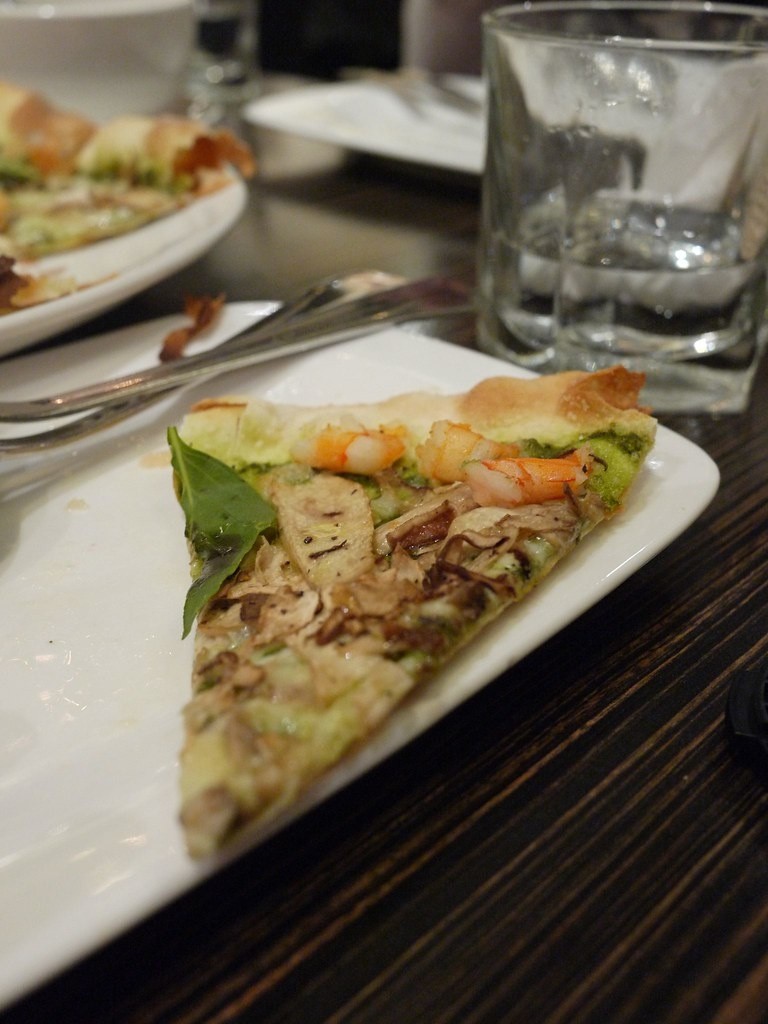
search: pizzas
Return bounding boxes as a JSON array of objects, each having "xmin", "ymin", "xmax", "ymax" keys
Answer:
[
  {"xmin": 0, "ymin": 84, "xmax": 258, "ymax": 320},
  {"xmin": 165, "ymin": 365, "xmax": 658, "ymax": 859}
]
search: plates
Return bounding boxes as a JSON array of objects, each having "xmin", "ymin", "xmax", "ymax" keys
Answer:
[
  {"xmin": 0, "ymin": 153, "xmax": 249, "ymax": 358},
  {"xmin": 241, "ymin": 71, "xmax": 487, "ymax": 174},
  {"xmin": 0, "ymin": 302, "xmax": 723, "ymax": 1020}
]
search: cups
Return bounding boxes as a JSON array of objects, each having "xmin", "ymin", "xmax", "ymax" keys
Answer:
[
  {"xmin": 474, "ymin": 0, "xmax": 768, "ymax": 415},
  {"xmin": 0, "ymin": 0, "xmax": 205, "ymax": 121}
]
